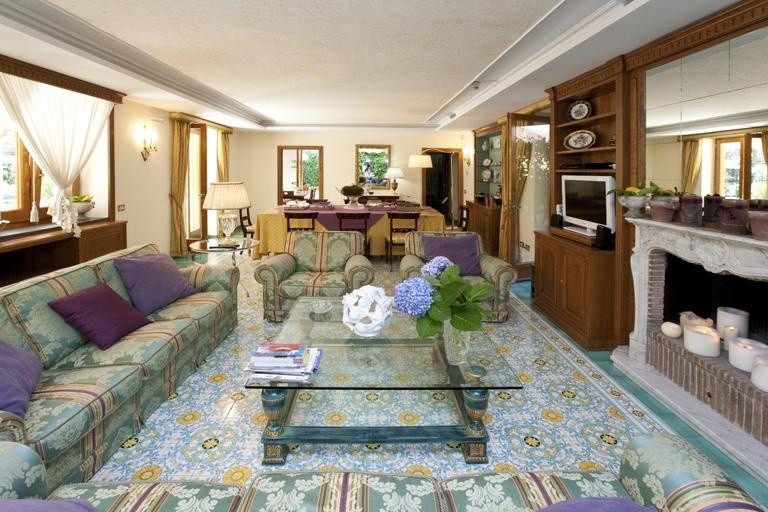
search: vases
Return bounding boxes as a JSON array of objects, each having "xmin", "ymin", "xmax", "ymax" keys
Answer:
[
  {"xmin": 721, "ymin": 194, "xmax": 768, "ymax": 240},
  {"xmin": 704, "ymin": 194, "xmax": 724, "ymax": 223},
  {"xmin": 442, "ymin": 318, "xmax": 474, "ymax": 365},
  {"xmin": 680, "ymin": 194, "xmax": 703, "ymax": 227}
]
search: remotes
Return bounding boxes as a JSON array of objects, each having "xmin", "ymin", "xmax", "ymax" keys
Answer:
[{"xmin": 209, "ymin": 245, "xmax": 239, "ymax": 249}]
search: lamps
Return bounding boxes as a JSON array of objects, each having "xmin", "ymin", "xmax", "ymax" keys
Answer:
[
  {"xmin": 140, "ymin": 129, "xmax": 159, "ymax": 162},
  {"xmin": 409, "ymin": 154, "xmax": 432, "ymax": 169},
  {"xmin": 465, "ymin": 153, "xmax": 470, "ymax": 166},
  {"xmin": 201, "ymin": 182, "xmax": 253, "ymax": 246},
  {"xmin": 384, "ymin": 168, "xmax": 405, "ymax": 195}
]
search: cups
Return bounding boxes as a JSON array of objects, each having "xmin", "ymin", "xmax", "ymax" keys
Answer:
[{"xmin": 649, "ymin": 195, "xmax": 748, "ymax": 235}]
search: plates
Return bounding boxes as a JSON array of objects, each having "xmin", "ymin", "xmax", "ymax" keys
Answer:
[
  {"xmin": 310, "ymin": 300, "xmax": 332, "ymax": 314},
  {"xmin": 567, "ymin": 101, "xmax": 590, "ymax": 122},
  {"xmin": 564, "ymin": 130, "xmax": 598, "ymax": 151},
  {"xmin": 483, "ymin": 159, "xmax": 491, "ymax": 166},
  {"xmin": 482, "ymin": 169, "xmax": 491, "ymax": 180}
]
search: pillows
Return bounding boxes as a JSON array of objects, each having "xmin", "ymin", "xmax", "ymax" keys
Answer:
[
  {"xmin": 114, "ymin": 253, "xmax": 202, "ymax": 317},
  {"xmin": 47, "ymin": 282, "xmax": 154, "ymax": 351},
  {"xmin": 1, "ymin": 336, "xmax": 45, "ymax": 419},
  {"xmin": 535, "ymin": 497, "xmax": 660, "ymax": 512},
  {"xmin": 4, "ymin": 500, "xmax": 97, "ymax": 512}
]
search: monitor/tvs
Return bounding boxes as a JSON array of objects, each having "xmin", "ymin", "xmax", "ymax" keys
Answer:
[{"xmin": 563, "ymin": 175, "xmax": 616, "ymax": 239}]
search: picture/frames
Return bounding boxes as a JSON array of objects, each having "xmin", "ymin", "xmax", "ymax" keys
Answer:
[{"xmin": 355, "ymin": 144, "xmax": 392, "ymax": 192}]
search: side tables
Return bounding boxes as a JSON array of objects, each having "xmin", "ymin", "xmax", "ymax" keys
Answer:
[{"xmin": 190, "ymin": 238, "xmax": 261, "ymax": 297}]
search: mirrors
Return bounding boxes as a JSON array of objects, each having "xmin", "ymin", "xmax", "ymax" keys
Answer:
[{"xmin": 644, "ymin": 26, "xmax": 768, "ymax": 226}]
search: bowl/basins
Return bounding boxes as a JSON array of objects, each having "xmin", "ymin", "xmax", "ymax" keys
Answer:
[
  {"xmin": 475, "ymin": 192, "xmax": 501, "ymax": 205},
  {"xmin": 0, "ymin": 220, "xmax": 10, "ymax": 232}
]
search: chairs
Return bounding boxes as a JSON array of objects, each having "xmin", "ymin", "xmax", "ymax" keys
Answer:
[
  {"xmin": 239, "ymin": 206, "xmax": 256, "ymax": 256},
  {"xmin": 254, "ymin": 231, "xmax": 518, "ymax": 324},
  {"xmin": 283, "ymin": 188, "xmax": 316, "ymax": 204},
  {"xmin": 447, "ymin": 206, "xmax": 470, "ymax": 234},
  {"xmin": 288, "ymin": 199, "xmax": 419, "ymax": 268}
]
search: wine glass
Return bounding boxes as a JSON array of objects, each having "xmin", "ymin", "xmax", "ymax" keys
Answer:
[{"xmin": 617, "ymin": 196, "xmax": 650, "ymax": 218}]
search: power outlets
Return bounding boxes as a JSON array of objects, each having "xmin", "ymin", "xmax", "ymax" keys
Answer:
[{"xmin": 519, "ymin": 241, "xmax": 530, "ymax": 251}]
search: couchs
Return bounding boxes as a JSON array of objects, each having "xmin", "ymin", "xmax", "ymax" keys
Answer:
[
  {"xmin": 2, "ymin": 432, "xmax": 764, "ymax": 512},
  {"xmin": 1, "ymin": 242, "xmax": 241, "ymax": 494}
]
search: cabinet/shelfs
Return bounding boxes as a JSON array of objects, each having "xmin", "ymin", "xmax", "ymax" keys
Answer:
[
  {"xmin": 532, "ymin": 71, "xmax": 630, "ymax": 352},
  {"xmin": 466, "ymin": 124, "xmax": 502, "ymax": 255}
]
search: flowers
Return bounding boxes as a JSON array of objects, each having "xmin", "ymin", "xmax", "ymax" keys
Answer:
[{"xmin": 393, "ymin": 256, "xmax": 496, "ymax": 339}]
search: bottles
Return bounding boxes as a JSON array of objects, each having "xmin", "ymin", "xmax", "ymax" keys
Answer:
[
  {"xmin": 556, "ymin": 202, "xmax": 562, "ymax": 216},
  {"xmin": 609, "ymin": 139, "xmax": 615, "ymax": 146},
  {"xmin": 482, "ymin": 141, "xmax": 490, "ymax": 151}
]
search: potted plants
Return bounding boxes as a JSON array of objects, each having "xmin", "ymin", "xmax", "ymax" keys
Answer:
[
  {"xmin": 607, "ymin": 181, "xmax": 680, "ymax": 222},
  {"xmin": 341, "ymin": 185, "xmax": 364, "ymax": 210},
  {"xmin": 70, "ymin": 192, "xmax": 95, "ymax": 222}
]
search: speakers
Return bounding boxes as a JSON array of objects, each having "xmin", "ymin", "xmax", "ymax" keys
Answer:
[
  {"xmin": 596, "ymin": 224, "xmax": 615, "ymax": 251},
  {"xmin": 551, "ymin": 214, "xmax": 563, "ymax": 228}
]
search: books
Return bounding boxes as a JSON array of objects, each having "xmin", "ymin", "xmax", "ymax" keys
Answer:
[{"xmin": 244, "ymin": 344, "xmax": 323, "ymax": 383}]
